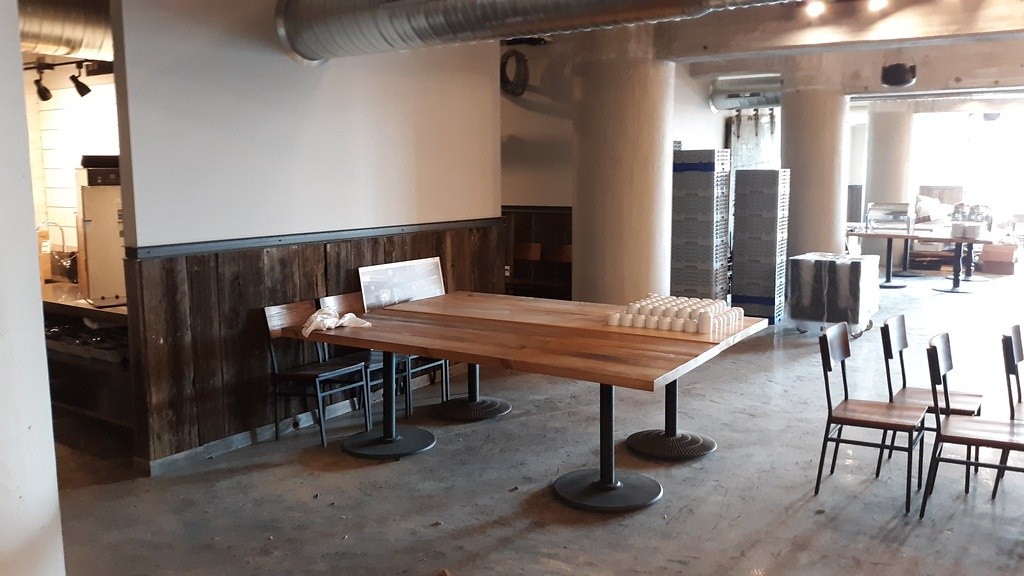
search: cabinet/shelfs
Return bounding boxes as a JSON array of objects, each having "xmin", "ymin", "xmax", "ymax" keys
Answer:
[{"xmin": 43, "ymin": 280, "xmax": 137, "ymax": 433}]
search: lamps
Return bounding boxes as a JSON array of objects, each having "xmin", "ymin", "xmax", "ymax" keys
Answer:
[
  {"xmin": 69, "ymin": 62, "xmax": 91, "ymax": 97},
  {"xmin": 34, "ymin": 68, "xmax": 53, "ymax": 102}
]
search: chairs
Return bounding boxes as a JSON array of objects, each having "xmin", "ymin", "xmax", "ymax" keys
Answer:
[
  {"xmin": 990, "ymin": 325, "xmax": 1024, "ymax": 500},
  {"xmin": 262, "ymin": 299, "xmax": 371, "ymax": 448},
  {"xmin": 317, "ymin": 292, "xmax": 450, "ymax": 431},
  {"xmin": 920, "ymin": 333, "xmax": 1024, "ymax": 517},
  {"xmin": 874, "ymin": 315, "xmax": 984, "ymax": 495},
  {"xmin": 813, "ymin": 322, "xmax": 929, "ymax": 513}
]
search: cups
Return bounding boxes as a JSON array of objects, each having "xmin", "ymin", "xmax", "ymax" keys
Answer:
[{"xmin": 607, "ymin": 293, "xmax": 744, "ymax": 334}]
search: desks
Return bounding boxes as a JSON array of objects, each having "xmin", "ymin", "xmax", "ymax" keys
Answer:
[
  {"xmin": 849, "ymin": 228, "xmax": 996, "ymax": 292},
  {"xmin": 284, "ymin": 289, "xmax": 769, "ymax": 513}
]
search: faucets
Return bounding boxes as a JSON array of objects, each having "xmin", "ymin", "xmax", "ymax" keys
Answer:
[{"xmin": 35, "ymin": 220, "xmax": 65, "ymax": 257}]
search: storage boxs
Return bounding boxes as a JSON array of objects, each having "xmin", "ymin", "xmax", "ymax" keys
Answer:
[
  {"xmin": 669, "ymin": 150, "xmax": 789, "ymax": 323},
  {"xmin": 981, "ymin": 243, "xmax": 1017, "ymax": 275}
]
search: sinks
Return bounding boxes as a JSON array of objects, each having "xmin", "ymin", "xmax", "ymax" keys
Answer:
[{"xmin": 41, "ymin": 282, "xmax": 84, "ymax": 304}]
